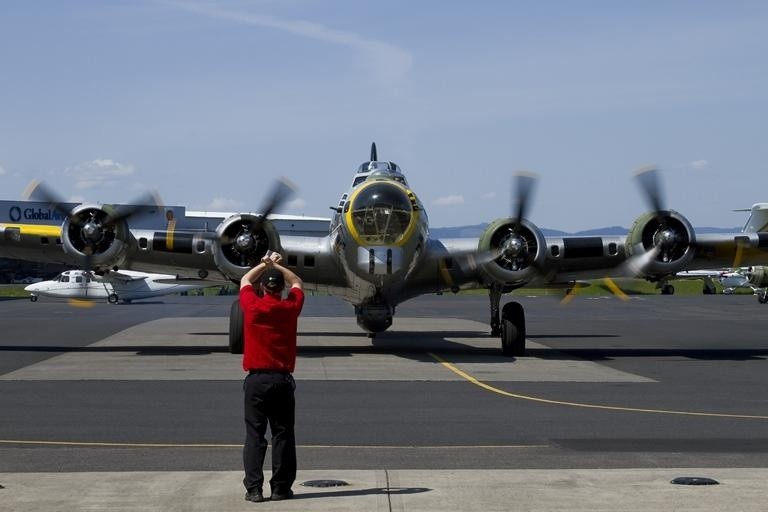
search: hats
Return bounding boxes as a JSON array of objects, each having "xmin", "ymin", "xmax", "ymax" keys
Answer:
[{"xmin": 261, "ymin": 269, "xmax": 285, "ymax": 283}]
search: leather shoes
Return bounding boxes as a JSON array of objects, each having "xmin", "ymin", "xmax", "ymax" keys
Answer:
[
  {"xmin": 271, "ymin": 488, "xmax": 294, "ymax": 500},
  {"xmin": 245, "ymin": 491, "xmax": 263, "ymax": 502}
]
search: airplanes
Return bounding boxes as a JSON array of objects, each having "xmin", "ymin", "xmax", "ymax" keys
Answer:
[
  {"xmin": 677, "ymin": 265, "xmax": 768, "ymax": 306},
  {"xmin": 0, "ymin": 136, "xmax": 768, "ymax": 363},
  {"xmin": 22, "ymin": 265, "xmax": 227, "ymax": 308}
]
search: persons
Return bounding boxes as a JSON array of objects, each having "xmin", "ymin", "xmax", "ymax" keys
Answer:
[{"xmin": 240, "ymin": 251, "xmax": 305, "ymax": 502}]
